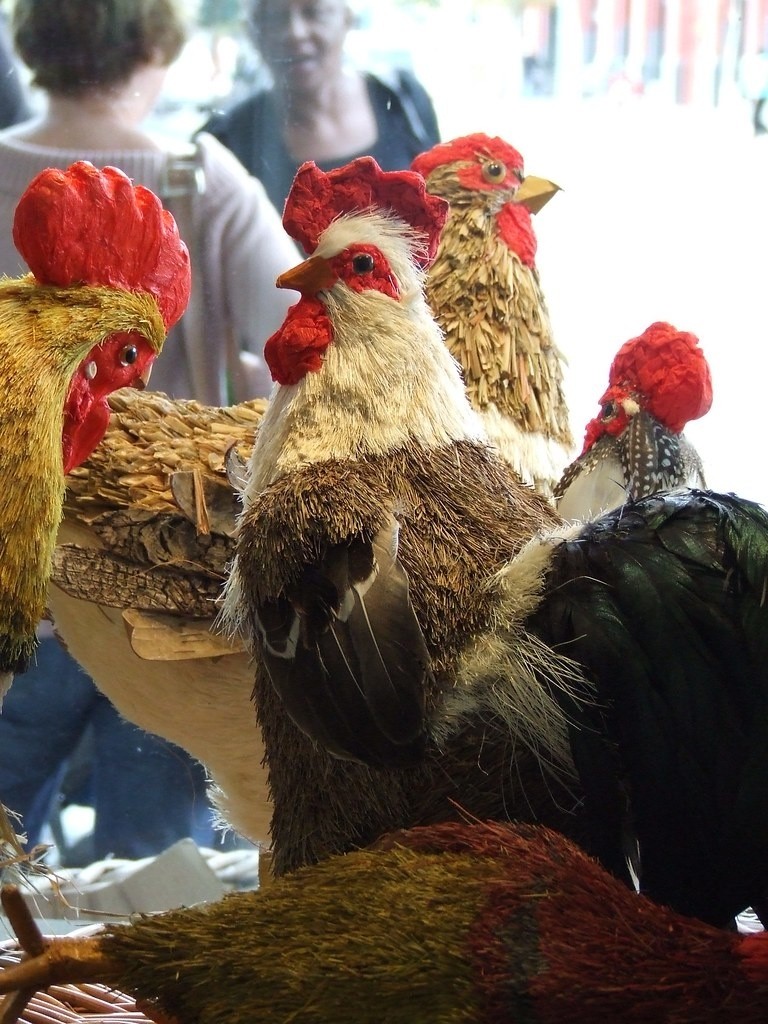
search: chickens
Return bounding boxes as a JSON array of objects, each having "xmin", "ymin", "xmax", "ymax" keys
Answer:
[{"xmin": 1, "ymin": 132, "xmax": 768, "ymax": 1024}]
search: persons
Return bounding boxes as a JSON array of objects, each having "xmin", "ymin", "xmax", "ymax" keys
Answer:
[
  {"xmin": 0, "ymin": 0, "xmax": 307, "ymax": 872},
  {"xmin": 192, "ymin": 0, "xmax": 442, "ymax": 261}
]
